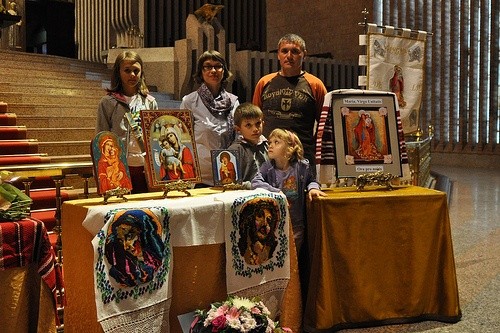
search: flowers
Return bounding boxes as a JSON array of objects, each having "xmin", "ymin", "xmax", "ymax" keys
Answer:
[{"xmin": 193, "ymin": 294, "xmax": 293, "ymax": 333}]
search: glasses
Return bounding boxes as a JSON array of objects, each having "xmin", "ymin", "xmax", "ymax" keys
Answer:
[{"xmin": 202, "ymin": 65, "xmax": 224, "ymax": 71}]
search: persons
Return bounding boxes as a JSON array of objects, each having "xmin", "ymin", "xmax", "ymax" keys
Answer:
[
  {"xmin": 179, "ymin": 49, "xmax": 241, "ymax": 189},
  {"xmin": 226, "ymin": 102, "xmax": 270, "ymax": 182},
  {"xmin": 253, "ymin": 33, "xmax": 327, "ymax": 177},
  {"xmin": 251, "ymin": 128, "xmax": 328, "ymax": 253},
  {"xmin": 95, "ymin": 50, "xmax": 159, "ymax": 195}
]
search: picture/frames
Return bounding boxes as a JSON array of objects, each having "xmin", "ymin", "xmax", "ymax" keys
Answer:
[{"xmin": 330, "ymin": 94, "xmax": 403, "ymax": 179}]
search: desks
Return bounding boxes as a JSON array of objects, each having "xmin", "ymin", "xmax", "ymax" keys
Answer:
[
  {"xmin": 303, "ymin": 183, "xmax": 462, "ymax": 333},
  {"xmin": 0, "ymin": 215, "xmax": 67, "ymax": 333},
  {"xmin": 61, "ymin": 188, "xmax": 301, "ymax": 333}
]
